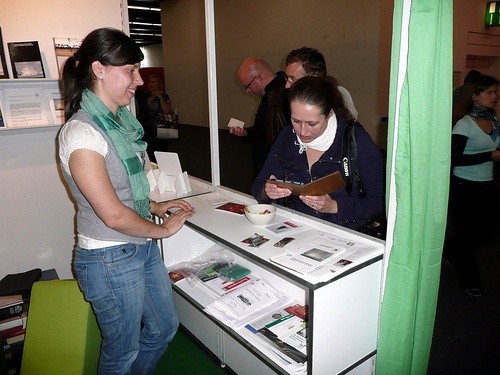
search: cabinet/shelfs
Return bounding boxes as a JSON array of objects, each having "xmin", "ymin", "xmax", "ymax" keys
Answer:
[{"xmin": 155, "ymin": 187, "xmax": 386, "ymax": 375}]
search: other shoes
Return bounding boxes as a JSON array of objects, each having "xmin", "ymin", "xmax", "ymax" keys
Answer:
[
  {"xmin": 463, "ymin": 284, "xmax": 485, "ymax": 298},
  {"xmin": 443, "ymin": 255, "xmax": 460, "ymax": 268}
]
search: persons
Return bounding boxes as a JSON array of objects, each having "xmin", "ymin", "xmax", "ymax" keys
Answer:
[
  {"xmin": 229, "ymin": 47, "xmax": 383, "ymax": 230},
  {"xmin": 56, "ymin": 27, "xmax": 197, "ymax": 375},
  {"xmin": 451, "ymin": 69, "xmax": 500, "ymax": 297},
  {"xmin": 144, "ymin": 74, "xmax": 171, "ymax": 121}
]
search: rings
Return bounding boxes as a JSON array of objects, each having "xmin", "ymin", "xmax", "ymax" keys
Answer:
[{"xmin": 312, "ymin": 203, "xmax": 315, "ymax": 207}]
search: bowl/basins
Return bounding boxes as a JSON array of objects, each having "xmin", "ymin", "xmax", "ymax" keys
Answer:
[{"xmin": 243, "ymin": 204, "xmax": 277, "ymax": 225}]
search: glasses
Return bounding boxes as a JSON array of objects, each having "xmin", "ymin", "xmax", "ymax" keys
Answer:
[
  {"xmin": 285, "ymin": 76, "xmax": 297, "ymax": 85},
  {"xmin": 245, "ymin": 76, "xmax": 256, "ymax": 90}
]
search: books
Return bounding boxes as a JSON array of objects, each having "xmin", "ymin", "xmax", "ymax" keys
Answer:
[{"xmin": 266, "ymin": 171, "xmax": 345, "ymax": 197}]
